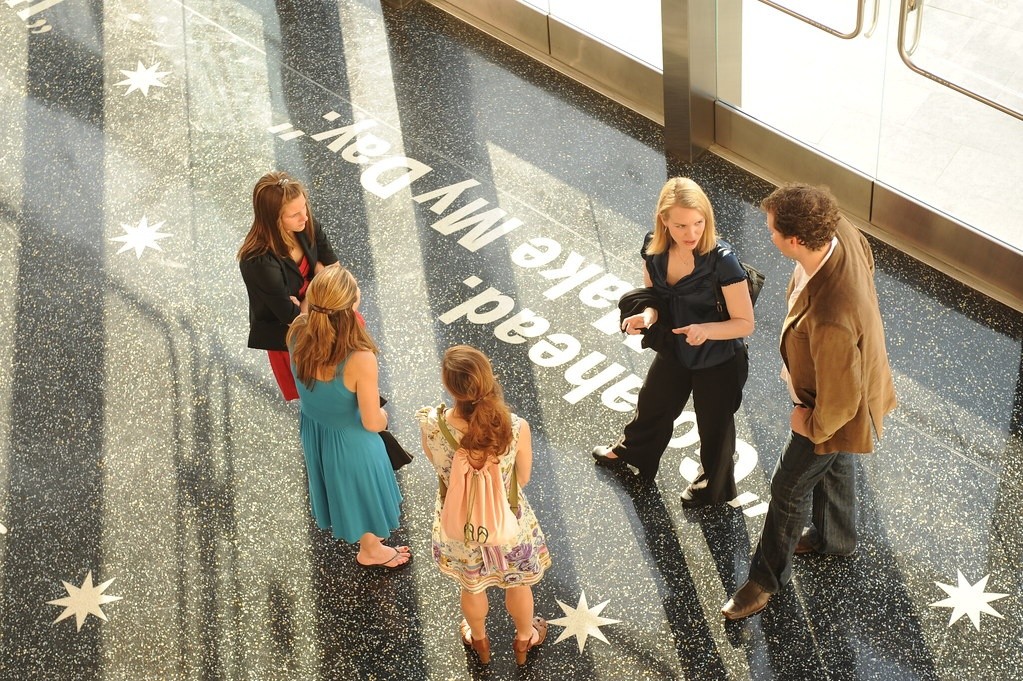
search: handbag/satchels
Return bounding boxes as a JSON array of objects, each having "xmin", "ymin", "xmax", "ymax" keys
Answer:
[{"xmin": 713, "ymin": 248, "xmax": 767, "ymax": 323}]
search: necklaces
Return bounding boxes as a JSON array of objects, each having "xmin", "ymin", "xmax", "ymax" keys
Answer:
[{"xmin": 676, "ymin": 245, "xmax": 693, "ymax": 264}]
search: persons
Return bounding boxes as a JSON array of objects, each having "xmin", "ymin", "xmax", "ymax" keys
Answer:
[
  {"xmin": 236, "ymin": 171, "xmax": 341, "ymax": 402},
  {"xmin": 286, "ymin": 266, "xmax": 411, "ymax": 570},
  {"xmin": 722, "ymin": 182, "xmax": 897, "ymax": 621},
  {"xmin": 414, "ymin": 345, "xmax": 552, "ymax": 664},
  {"xmin": 592, "ymin": 177, "xmax": 754, "ymax": 506}
]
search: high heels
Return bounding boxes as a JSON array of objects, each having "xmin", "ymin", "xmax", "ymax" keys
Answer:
[
  {"xmin": 513, "ymin": 616, "xmax": 546, "ymax": 665},
  {"xmin": 680, "ymin": 485, "xmax": 702, "ymax": 507},
  {"xmin": 594, "ymin": 445, "xmax": 657, "ymax": 482},
  {"xmin": 460, "ymin": 618, "xmax": 491, "ymax": 663}
]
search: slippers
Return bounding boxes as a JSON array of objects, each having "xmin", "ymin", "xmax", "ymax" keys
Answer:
[{"xmin": 355, "ymin": 547, "xmax": 413, "ymax": 570}]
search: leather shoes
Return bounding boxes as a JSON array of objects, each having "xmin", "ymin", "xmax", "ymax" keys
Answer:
[
  {"xmin": 721, "ymin": 578, "xmax": 771, "ymax": 619},
  {"xmin": 793, "ymin": 526, "xmax": 856, "ymax": 557}
]
